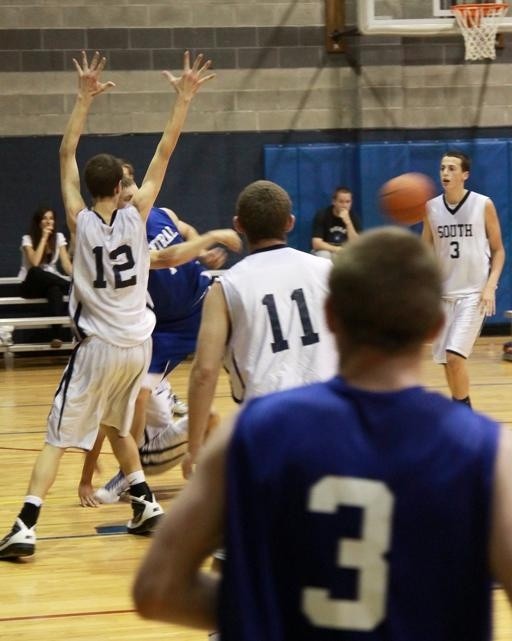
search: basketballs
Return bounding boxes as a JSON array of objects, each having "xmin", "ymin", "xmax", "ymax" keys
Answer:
[{"xmin": 381, "ymin": 172, "xmax": 431, "ymax": 225}]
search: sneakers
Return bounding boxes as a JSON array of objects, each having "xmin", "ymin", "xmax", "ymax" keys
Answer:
[
  {"xmin": 93, "ymin": 468, "xmax": 132, "ymax": 504},
  {"xmin": 52, "ymin": 338, "xmax": 61, "ymax": 349},
  {"xmin": 127, "ymin": 492, "xmax": 165, "ymax": 533},
  {"xmin": 0, "ymin": 516, "xmax": 37, "ymax": 560}
]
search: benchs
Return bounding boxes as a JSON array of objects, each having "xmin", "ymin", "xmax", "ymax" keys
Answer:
[{"xmin": 0, "ymin": 277, "xmax": 80, "ymax": 370}]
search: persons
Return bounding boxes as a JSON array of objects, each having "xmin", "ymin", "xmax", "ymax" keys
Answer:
[
  {"xmin": 93, "ymin": 179, "xmax": 244, "ymax": 505},
  {"xmin": 133, "ymin": 226, "xmax": 511, "ymax": 641},
  {"xmin": 413, "ymin": 152, "xmax": 505, "ymax": 414},
  {"xmin": 13, "ymin": 205, "xmax": 74, "ymax": 350},
  {"xmin": 121, "ymin": 164, "xmax": 135, "ymax": 181},
  {"xmin": 77, "ymin": 372, "xmax": 223, "ymax": 508},
  {"xmin": 181, "ymin": 180, "xmax": 339, "ymax": 481},
  {"xmin": 310, "ymin": 188, "xmax": 361, "ymax": 265},
  {"xmin": 1, "ymin": 48, "xmax": 225, "ymax": 562}
]
